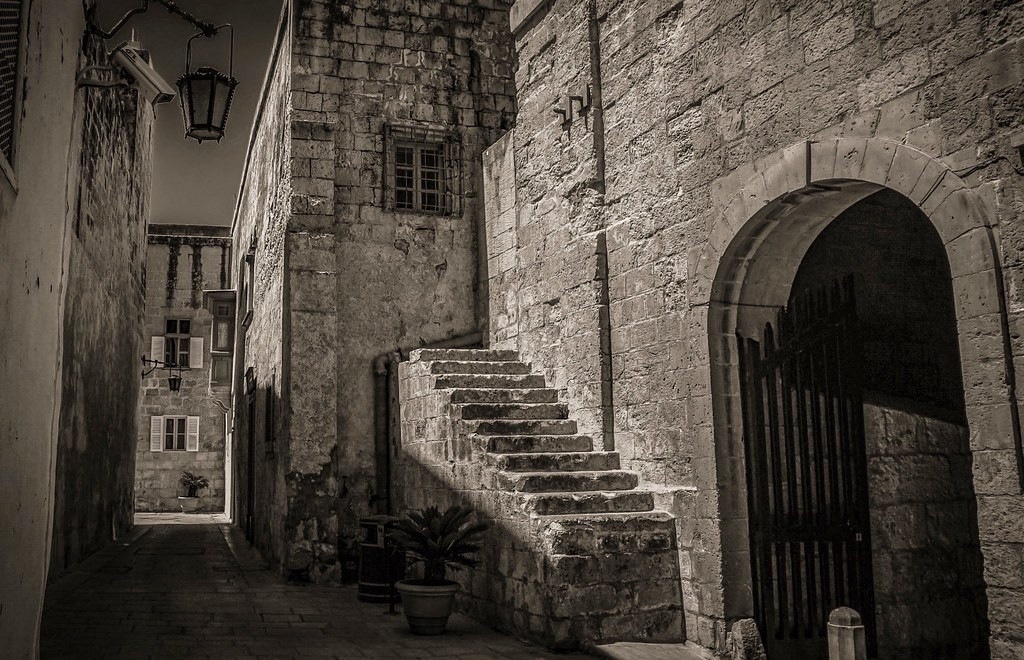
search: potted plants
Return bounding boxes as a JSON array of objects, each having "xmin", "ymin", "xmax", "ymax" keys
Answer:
[
  {"xmin": 177, "ymin": 471, "xmax": 210, "ymax": 513},
  {"xmin": 388, "ymin": 502, "xmax": 490, "ymax": 637}
]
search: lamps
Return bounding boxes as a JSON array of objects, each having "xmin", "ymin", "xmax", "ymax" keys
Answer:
[
  {"xmin": 139, "ymin": 354, "xmax": 183, "ymax": 395},
  {"xmin": 82, "ymin": 0, "xmax": 242, "ymax": 144}
]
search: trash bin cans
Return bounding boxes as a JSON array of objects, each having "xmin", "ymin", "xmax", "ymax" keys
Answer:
[{"xmin": 357, "ymin": 515, "xmax": 405, "ymax": 603}]
